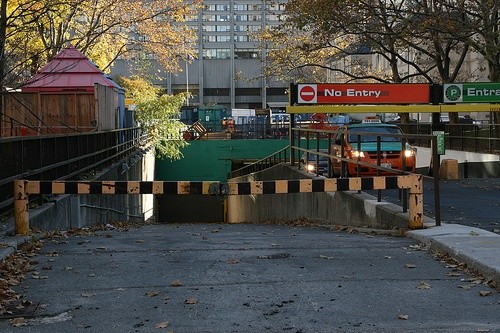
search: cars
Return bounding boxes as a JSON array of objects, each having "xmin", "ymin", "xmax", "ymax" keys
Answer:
[
  {"xmin": 301, "ymin": 149, "xmax": 330, "ymax": 178},
  {"xmin": 265, "ymin": 113, "xmax": 291, "ymax": 132},
  {"xmin": 331, "ymin": 118, "xmax": 417, "ymax": 182}
]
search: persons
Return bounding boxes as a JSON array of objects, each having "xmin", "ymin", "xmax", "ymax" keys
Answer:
[{"xmin": 221, "ymin": 116, "xmax": 235, "ymax": 134}]
